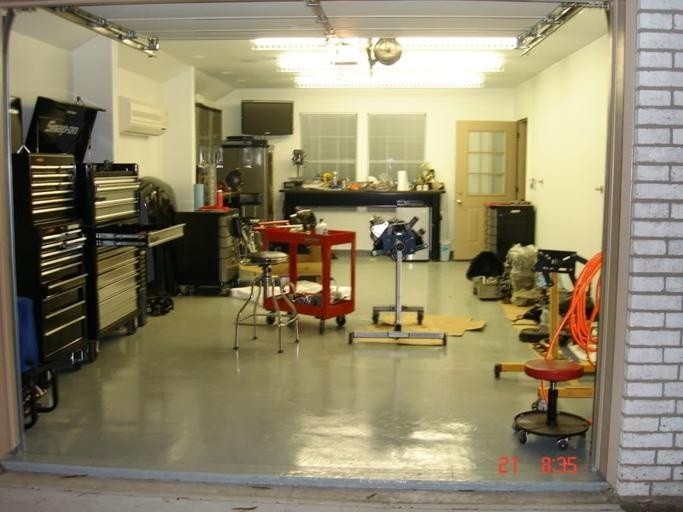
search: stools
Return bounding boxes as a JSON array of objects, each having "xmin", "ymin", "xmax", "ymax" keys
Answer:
[{"xmin": 511, "ymin": 359, "xmax": 591, "ymax": 451}]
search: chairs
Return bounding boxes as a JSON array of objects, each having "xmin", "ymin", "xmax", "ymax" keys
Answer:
[
  {"xmin": 234, "ymin": 192, "xmax": 263, "ymax": 266},
  {"xmin": 229, "ymin": 217, "xmax": 300, "ymax": 355}
]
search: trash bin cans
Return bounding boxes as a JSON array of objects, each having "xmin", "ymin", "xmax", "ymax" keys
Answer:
[{"xmin": 439, "ymin": 240, "xmax": 452, "ymax": 261}]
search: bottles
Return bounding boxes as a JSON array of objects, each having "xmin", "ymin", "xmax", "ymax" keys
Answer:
[
  {"xmin": 303, "ymin": 218, "xmax": 328, "ymax": 236},
  {"xmin": 316, "ymin": 169, "xmax": 346, "ymax": 189},
  {"xmin": 217, "ymin": 190, "xmax": 222, "ymax": 206}
]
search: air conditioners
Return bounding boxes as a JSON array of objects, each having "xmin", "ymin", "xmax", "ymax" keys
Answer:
[{"xmin": 117, "ymin": 97, "xmax": 168, "ymax": 140}]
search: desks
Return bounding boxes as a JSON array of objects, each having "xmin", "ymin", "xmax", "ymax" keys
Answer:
[{"xmin": 279, "ymin": 188, "xmax": 445, "ymax": 261}]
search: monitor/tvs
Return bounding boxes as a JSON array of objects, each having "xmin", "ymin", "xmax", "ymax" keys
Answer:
[{"xmin": 242, "ymin": 102, "xmax": 293, "ymax": 135}]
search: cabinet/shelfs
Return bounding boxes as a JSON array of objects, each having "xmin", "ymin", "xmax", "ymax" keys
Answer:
[
  {"xmin": 483, "ymin": 206, "xmax": 535, "ymax": 265},
  {"xmin": 79, "ymin": 160, "xmax": 185, "ymax": 352},
  {"xmin": 176, "ymin": 208, "xmax": 240, "ymax": 297}
]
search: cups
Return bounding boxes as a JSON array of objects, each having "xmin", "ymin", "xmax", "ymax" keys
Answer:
[
  {"xmin": 415, "ymin": 184, "xmax": 428, "ymax": 191},
  {"xmin": 193, "ymin": 183, "xmax": 204, "ymax": 209}
]
